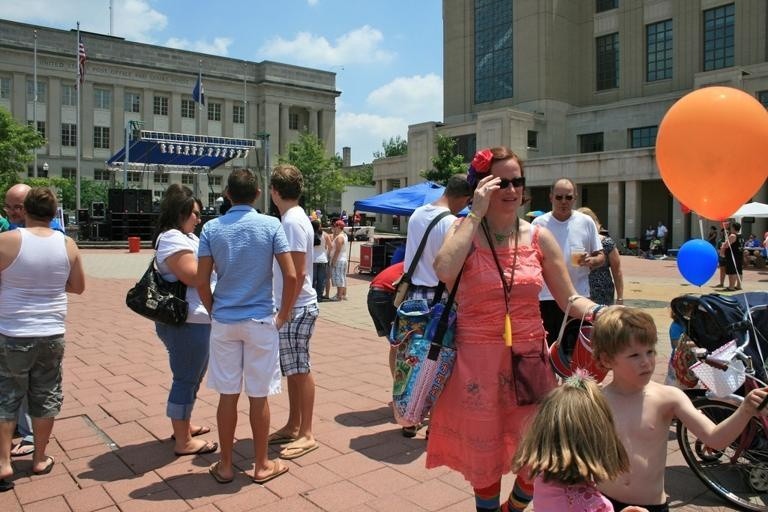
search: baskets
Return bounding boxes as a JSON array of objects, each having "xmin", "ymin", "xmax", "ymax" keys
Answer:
[{"xmin": 689, "ymin": 338, "xmax": 744, "ymax": 397}]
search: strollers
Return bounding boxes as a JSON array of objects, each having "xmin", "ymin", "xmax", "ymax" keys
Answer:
[{"xmin": 671, "ymin": 291, "xmax": 768, "ymax": 493}]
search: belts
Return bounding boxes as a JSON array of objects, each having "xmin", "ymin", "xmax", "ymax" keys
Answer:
[{"xmin": 369, "ymin": 286, "xmax": 389, "ymax": 291}]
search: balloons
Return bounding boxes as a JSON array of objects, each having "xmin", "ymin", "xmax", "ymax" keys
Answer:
[
  {"xmin": 676, "ymin": 238, "xmax": 719, "ymax": 292},
  {"xmin": 309, "ymin": 210, "xmax": 321, "ymax": 222},
  {"xmin": 654, "ymin": 84, "xmax": 768, "ymax": 413}
]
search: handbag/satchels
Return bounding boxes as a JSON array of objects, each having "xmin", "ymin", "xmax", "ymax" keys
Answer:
[
  {"xmin": 549, "ymin": 294, "xmax": 601, "ymax": 380},
  {"xmin": 125, "ymin": 233, "xmax": 191, "ymax": 330},
  {"xmin": 391, "ymin": 210, "xmax": 454, "ymax": 314}
]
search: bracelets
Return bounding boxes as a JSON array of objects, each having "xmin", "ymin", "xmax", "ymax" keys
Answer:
[
  {"xmin": 616, "ymin": 297, "xmax": 623, "ymax": 303},
  {"xmin": 616, "ymin": 297, "xmax": 626, "ymax": 305},
  {"xmin": 584, "ymin": 303, "xmax": 606, "ymax": 324}
]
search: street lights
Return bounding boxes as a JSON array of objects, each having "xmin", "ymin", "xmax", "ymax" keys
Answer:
[{"xmin": 43, "ymin": 160, "xmax": 49, "ymax": 179}]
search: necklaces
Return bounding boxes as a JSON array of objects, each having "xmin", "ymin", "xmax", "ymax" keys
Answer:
[
  {"xmin": 481, "ymin": 223, "xmax": 516, "ymax": 244},
  {"xmin": 479, "ymin": 215, "xmax": 520, "ymax": 349}
]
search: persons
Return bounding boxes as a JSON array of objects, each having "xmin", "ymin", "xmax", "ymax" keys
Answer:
[
  {"xmin": 531, "ymin": 177, "xmax": 606, "ymax": 386},
  {"xmin": 267, "ymin": 161, "xmax": 321, "ymax": 461},
  {"xmin": 762, "ymin": 231, "xmax": 768, "ymax": 269},
  {"xmin": 310, "ymin": 220, "xmax": 350, "ymax": 302},
  {"xmin": 367, "ymin": 260, "xmax": 407, "ymax": 380},
  {"xmin": 743, "ymin": 233, "xmax": 761, "ymax": 269},
  {"xmin": 402, "ymin": 174, "xmax": 475, "ymax": 438},
  {"xmin": 425, "ymin": 146, "xmax": 627, "ymax": 512},
  {"xmin": 152, "ymin": 182, "xmax": 218, "ymax": 457},
  {"xmin": 589, "ymin": 304, "xmax": 768, "ymax": 512},
  {"xmin": 663, "ymin": 303, "xmax": 703, "ymax": 392},
  {"xmin": 645, "ymin": 219, "xmax": 669, "ymax": 261},
  {"xmin": 0, "ymin": 183, "xmax": 85, "ymax": 480},
  {"xmin": 575, "ymin": 207, "xmax": 624, "ymax": 307},
  {"xmin": 508, "ymin": 367, "xmax": 650, "ymax": 511},
  {"xmin": 196, "ymin": 166, "xmax": 297, "ymax": 484},
  {"xmin": 708, "ymin": 219, "xmax": 744, "ymax": 292}
]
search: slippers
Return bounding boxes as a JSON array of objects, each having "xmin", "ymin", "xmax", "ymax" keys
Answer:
[
  {"xmin": 10, "ymin": 437, "xmax": 50, "ymax": 457},
  {"xmin": 209, "ymin": 461, "xmax": 234, "ymax": 484},
  {"xmin": 279, "ymin": 441, "xmax": 319, "ymax": 460},
  {"xmin": 268, "ymin": 429, "xmax": 297, "ymax": 445},
  {"xmin": 735, "ymin": 287, "xmax": 743, "ymax": 290},
  {"xmin": 251, "ymin": 459, "xmax": 290, "ymax": 484},
  {"xmin": 35, "ymin": 455, "xmax": 54, "ymax": 477},
  {"xmin": 722, "ymin": 287, "xmax": 737, "ymax": 292},
  {"xmin": 402, "ymin": 426, "xmax": 418, "ymax": 437},
  {"xmin": 0, "ymin": 476, "xmax": 16, "ymax": 493},
  {"xmin": 171, "ymin": 424, "xmax": 211, "ymax": 441},
  {"xmin": 387, "ymin": 264, "xmax": 459, "ymax": 429},
  {"xmin": 174, "ymin": 440, "xmax": 219, "ymax": 458}
]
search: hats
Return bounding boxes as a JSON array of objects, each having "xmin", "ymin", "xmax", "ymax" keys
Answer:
[{"xmin": 670, "ymin": 330, "xmax": 700, "ymax": 390}]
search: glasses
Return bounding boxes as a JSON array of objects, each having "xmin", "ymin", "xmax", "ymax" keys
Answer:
[
  {"xmin": 191, "ymin": 206, "xmax": 204, "ymax": 221},
  {"xmin": 552, "ymin": 191, "xmax": 575, "ymax": 202},
  {"xmin": 495, "ymin": 175, "xmax": 527, "ymax": 191},
  {"xmin": 2, "ymin": 205, "xmax": 27, "ymax": 213}
]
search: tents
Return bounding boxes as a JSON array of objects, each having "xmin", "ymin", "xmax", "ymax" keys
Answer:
[
  {"xmin": 347, "ymin": 178, "xmax": 470, "ymax": 274},
  {"xmin": 728, "ymin": 201, "xmax": 768, "ymax": 218}
]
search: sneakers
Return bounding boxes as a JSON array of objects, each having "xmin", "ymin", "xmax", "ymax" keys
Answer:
[{"xmin": 329, "ymin": 294, "xmax": 346, "ymax": 301}]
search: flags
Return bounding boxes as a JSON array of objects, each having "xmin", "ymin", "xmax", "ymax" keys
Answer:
[
  {"xmin": 192, "ymin": 73, "xmax": 205, "ymax": 107},
  {"xmin": 77, "ymin": 33, "xmax": 88, "ymax": 86}
]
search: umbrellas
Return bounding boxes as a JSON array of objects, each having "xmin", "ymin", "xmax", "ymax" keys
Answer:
[{"xmin": 525, "ymin": 210, "xmax": 547, "ymax": 217}]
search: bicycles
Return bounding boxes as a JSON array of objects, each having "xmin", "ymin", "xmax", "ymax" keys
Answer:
[{"xmin": 676, "ymin": 304, "xmax": 767, "ymax": 511}]
